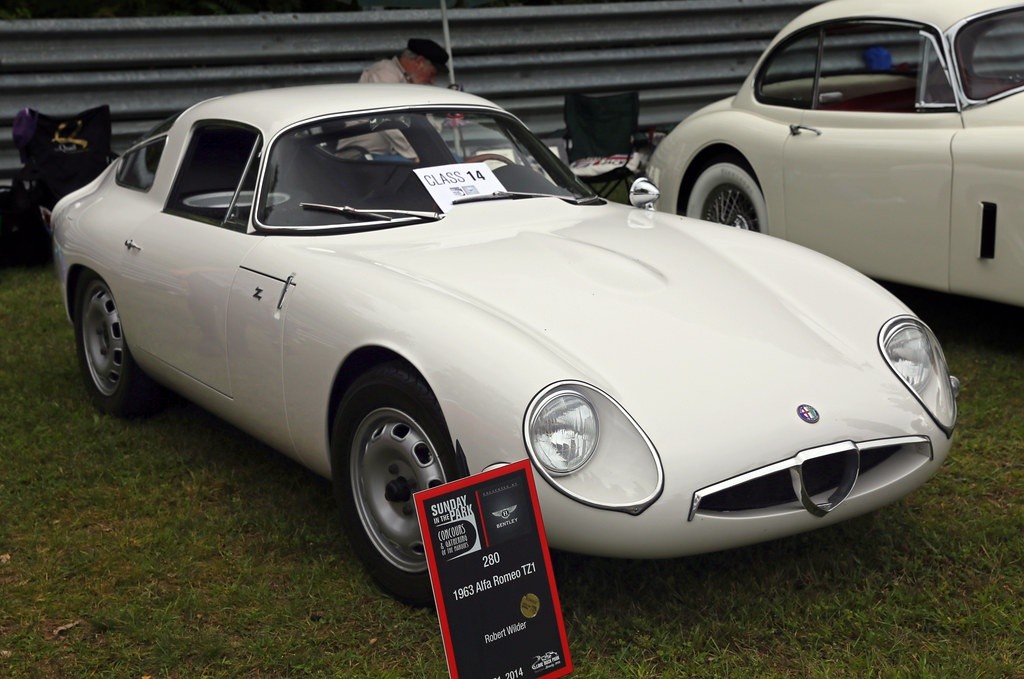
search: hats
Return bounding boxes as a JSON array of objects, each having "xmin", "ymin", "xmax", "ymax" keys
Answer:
[
  {"xmin": 865, "ymin": 47, "xmax": 891, "ymax": 72},
  {"xmin": 408, "ymin": 38, "xmax": 450, "ymax": 74}
]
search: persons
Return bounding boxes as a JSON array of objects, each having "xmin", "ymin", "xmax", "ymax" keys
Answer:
[{"xmin": 337, "ymin": 38, "xmax": 449, "ymax": 162}]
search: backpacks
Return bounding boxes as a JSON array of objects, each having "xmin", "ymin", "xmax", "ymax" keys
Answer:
[{"xmin": 0, "ymin": 175, "xmax": 52, "ymax": 266}]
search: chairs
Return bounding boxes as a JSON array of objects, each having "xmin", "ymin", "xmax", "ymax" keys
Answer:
[
  {"xmin": 560, "ymin": 89, "xmax": 669, "ymax": 199},
  {"xmin": 10, "ymin": 104, "xmax": 119, "ymax": 247}
]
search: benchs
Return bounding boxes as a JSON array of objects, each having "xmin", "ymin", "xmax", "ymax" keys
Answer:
[
  {"xmin": 762, "ymin": 74, "xmax": 938, "ymax": 105},
  {"xmin": 819, "ymin": 78, "xmax": 1024, "ymax": 113}
]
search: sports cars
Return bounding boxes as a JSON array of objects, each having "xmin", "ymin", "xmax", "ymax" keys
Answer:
[
  {"xmin": 643, "ymin": 0, "xmax": 1023, "ymax": 309},
  {"xmin": 48, "ymin": 78, "xmax": 961, "ymax": 613}
]
